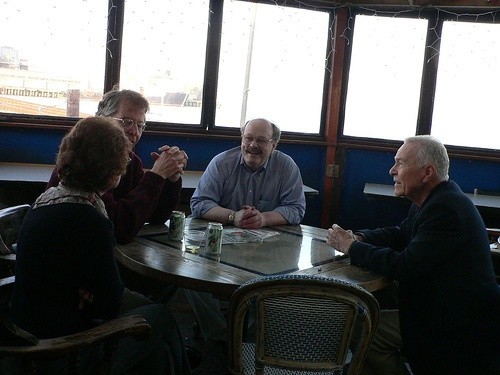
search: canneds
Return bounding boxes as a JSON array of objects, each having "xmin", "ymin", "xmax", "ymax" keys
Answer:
[
  {"xmin": 168, "ymin": 211, "xmax": 185, "ymax": 241},
  {"xmin": 204, "ymin": 222, "xmax": 224, "ymax": 255}
]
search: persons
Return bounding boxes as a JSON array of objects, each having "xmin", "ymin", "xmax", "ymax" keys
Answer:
[
  {"xmin": 190, "ymin": 118, "xmax": 307, "ymax": 227},
  {"xmin": 46, "ymin": 89, "xmax": 189, "ymax": 300},
  {"xmin": 328, "ymin": 135, "xmax": 500, "ymax": 375},
  {"xmin": 14, "ymin": 117, "xmax": 186, "ymax": 375}
]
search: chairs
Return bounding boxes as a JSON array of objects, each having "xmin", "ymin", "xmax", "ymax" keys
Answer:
[
  {"xmin": 228, "ymin": 273, "xmax": 380, "ymax": 375},
  {"xmin": 0, "ymin": 274, "xmax": 155, "ymax": 375}
]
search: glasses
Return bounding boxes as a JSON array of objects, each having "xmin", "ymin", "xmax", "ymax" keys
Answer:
[
  {"xmin": 241, "ymin": 135, "xmax": 276, "ymax": 147},
  {"xmin": 109, "ymin": 117, "xmax": 146, "ymax": 131}
]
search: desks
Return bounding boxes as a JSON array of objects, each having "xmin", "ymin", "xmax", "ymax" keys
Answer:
[
  {"xmin": 0, "ymin": 161, "xmax": 319, "ymax": 199},
  {"xmin": 362, "ymin": 182, "xmax": 500, "ymax": 210},
  {"xmin": 114, "ymin": 218, "xmax": 386, "ymax": 375}
]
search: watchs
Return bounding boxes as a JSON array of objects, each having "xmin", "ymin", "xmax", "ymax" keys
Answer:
[{"xmin": 229, "ymin": 211, "xmax": 235, "ymax": 224}]
